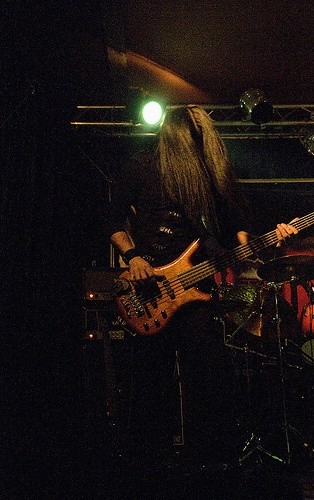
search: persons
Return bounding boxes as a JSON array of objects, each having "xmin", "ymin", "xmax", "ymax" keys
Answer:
[{"xmin": 103, "ymin": 105, "xmax": 299, "ymax": 452}]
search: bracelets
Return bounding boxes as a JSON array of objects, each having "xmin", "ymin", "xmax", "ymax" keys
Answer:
[{"xmin": 121, "ymin": 249, "xmax": 137, "ymax": 263}]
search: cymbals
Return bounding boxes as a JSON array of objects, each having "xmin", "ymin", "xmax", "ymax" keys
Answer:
[
  {"xmin": 257, "ymin": 253, "xmax": 314, "ymax": 284},
  {"xmin": 226, "ymin": 285, "xmax": 299, "ymax": 344}
]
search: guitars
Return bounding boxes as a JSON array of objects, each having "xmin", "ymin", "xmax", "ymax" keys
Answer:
[{"xmin": 110, "ymin": 210, "xmax": 314, "ymax": 337}]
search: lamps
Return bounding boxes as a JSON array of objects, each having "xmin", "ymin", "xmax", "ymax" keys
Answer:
[
  {"xmin": 240, "ymin": 88, "xmax": 273, "ymax": 126},
  {"xmin": 132, "ymin": 94, "xmax": 167, "ymax": 134}
]
